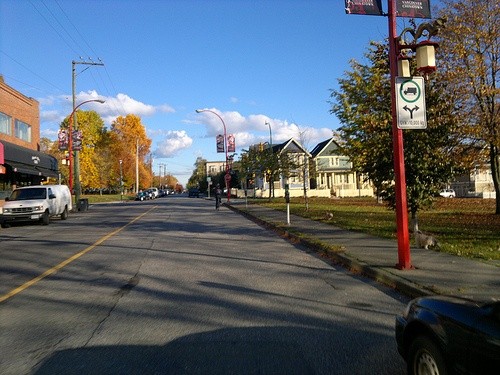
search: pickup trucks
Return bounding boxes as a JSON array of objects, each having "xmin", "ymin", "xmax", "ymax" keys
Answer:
[{"xmin": 2, "ymin": 184, "xmax": 73, "ymax": 228}]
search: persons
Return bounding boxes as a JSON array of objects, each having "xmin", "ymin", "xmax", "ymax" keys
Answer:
[{"xmin": 215, "ymin": 192, "xmax": 222, "ymax": 213}]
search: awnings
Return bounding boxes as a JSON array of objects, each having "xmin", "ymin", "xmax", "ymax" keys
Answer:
[
  {"xmin": 0, "ymin": 165, "xmax": 6, "ymax": 174},
  {"xmin": 5, "ymin": 159, "xmax": 66, "ymax": 179}
]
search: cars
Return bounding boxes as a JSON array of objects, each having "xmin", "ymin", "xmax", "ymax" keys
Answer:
[
  {"xmin": 395, "ymin": 294, "xmax": 500, "ymax": 375},
  {"xmin": 135, "ymin": 192, "xmax": 150, "ymax": 201},
  {"xmin": 159, "ymin": 189, "xmax": 174, "ymax": 198}
]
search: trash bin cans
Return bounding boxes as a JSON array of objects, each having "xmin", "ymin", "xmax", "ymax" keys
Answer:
[{"xmin": 79, "ymin": 199, "xmax": 88, "ymax": 210}]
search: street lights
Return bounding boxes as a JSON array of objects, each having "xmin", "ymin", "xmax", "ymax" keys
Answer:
[
  {"xmin": 119, "ymin": 158, "xmax": 124, "ymax": 201},
  {"xmin": 265, "ymin": 122, "xmax": 275, "ymax": 199},
  {"xmin": 196, "ymin": 109, "xmax": 231, "ymax": 202},
  {"xmin": 387, "ymin": 0, "xmax": 440, "ymax": 271},
  {"xmin": 68, "ymin": 99, "xmax": 105, "ymax": 194}
]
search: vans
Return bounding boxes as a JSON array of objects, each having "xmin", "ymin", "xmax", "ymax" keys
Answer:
[
  {"xmin": 146, "ymin": 188, "xmax": 159, "ymax": 199},
  {"xmin": 439, "ymin": 189, "xmax": 456, "ymax": 199}
]
